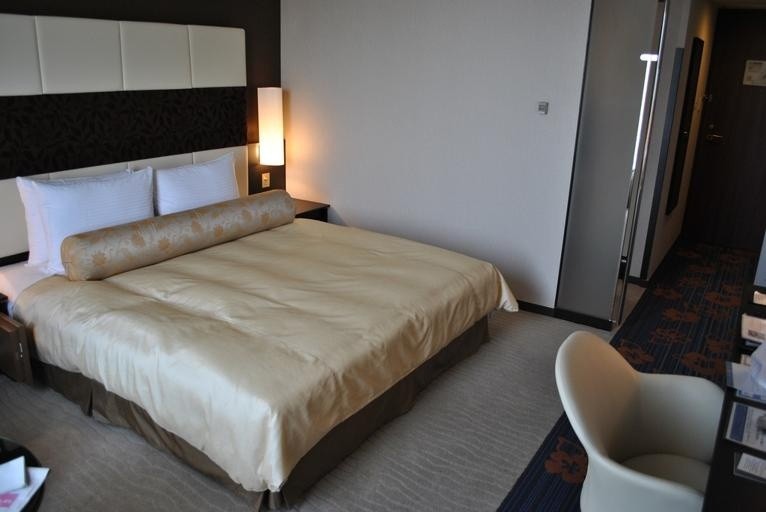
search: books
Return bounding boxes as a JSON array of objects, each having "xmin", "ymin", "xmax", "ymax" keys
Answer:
[
  {"xmin": 724, "ymin": 346, "xmax": 766, "ymax": 403},
  {"xmin": 741, "ymin": 313, "xmax": 766, "ymax": 346},
  {"xmin": 0, "ymin": 466, "xmax": 51, "ymax": 511},
  {"xmin": 0, "ymin": 455, "xmax": 29, "ymax": 494},
  {"xmin": 722, "ymin": 399, "xmax": 766, "ymax": 457}
]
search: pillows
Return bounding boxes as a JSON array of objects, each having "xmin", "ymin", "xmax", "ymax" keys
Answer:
[
  {"xmin": 16, "ymin": 166, "xmax": 134, "ymax": 268},
  {"xmin": 153, "ymin": 151, "xmax": 242, "ymax": 217},
  {"xmin": 31, "ymin": 166, "xmax": 153, "ymax": 271},
  {"xmin": 61, "ymin": 190, "xmax": 296, "ymax": 282}
]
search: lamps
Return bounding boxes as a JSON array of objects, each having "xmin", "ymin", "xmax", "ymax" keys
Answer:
[{"xmin": 256, "ymin": 87, "xmax": 286, "ymax": 167}]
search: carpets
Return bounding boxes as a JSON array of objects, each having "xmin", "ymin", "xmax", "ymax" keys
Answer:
[{"xmin": 0, "ymin": 310, "xmax": 616, "ymax": 512}]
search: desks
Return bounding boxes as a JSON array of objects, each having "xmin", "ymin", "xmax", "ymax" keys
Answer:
[
  {"xmin": 703, "ymin": 285, "xmax": 766, "ymax": 512},
  {"xmin": 0, "ymin": 438, "xmax": 45, "ymax": 511}
]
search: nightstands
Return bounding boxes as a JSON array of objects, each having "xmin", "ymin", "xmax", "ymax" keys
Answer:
[
  {"xmin": 0, "ymin": 291, "xmax": 34, "ymax": 388},
  {"xmin": 292, "ymin": 197, "xmax": 330, "ymax": 222}
]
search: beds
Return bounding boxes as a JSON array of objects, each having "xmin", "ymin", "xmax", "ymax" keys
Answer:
[{"xmin": 0, "ymin": 214, "xmax": 521, "ymax": 511}]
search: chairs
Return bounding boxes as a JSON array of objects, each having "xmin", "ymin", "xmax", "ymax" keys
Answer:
[{"xmin": 556, "ymin": 330, "xmax": 726, "ymax": 512}]
